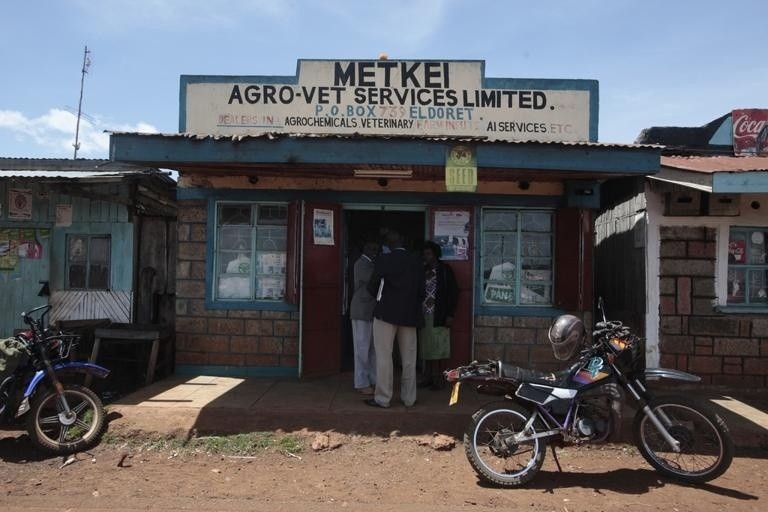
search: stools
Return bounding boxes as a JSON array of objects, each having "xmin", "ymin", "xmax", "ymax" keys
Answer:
[{"xmin": 84, "ymin": 324, "xmax": 172, "ymax": 388}]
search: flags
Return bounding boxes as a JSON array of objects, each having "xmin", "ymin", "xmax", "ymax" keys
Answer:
[{"xmin": 442, "ymin": 140, "xmax": 480, "ymax": 193}]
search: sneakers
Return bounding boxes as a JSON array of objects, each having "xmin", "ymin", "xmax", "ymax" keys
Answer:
[{"xmin": 356, "ymin": 378, "xmax": 446, "ymax": 408}]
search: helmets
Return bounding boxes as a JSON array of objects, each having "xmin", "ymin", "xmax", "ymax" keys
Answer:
[{"xmin": 545, "ymin": 312, "xmax": 587, "ymax": 361}]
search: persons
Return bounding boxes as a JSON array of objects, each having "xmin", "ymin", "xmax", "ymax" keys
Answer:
[
  {"xmin": 349, "ymin": 239, "xmax": 384, "ymax": 395},
  {"xmin": 362, "ymin": 229, "xmax": 426, "ymax": 409},
  {"xmin": 417, "ymin": 239, "xmax": 459, "ymax": 391}
]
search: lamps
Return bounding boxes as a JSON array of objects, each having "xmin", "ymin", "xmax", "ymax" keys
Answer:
[{"xmin": 353, "ymin": 169, "xmax": 413, "ymax": 180}]
